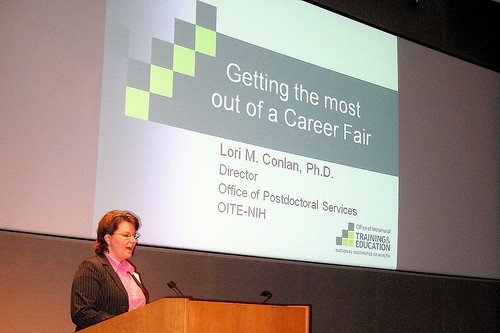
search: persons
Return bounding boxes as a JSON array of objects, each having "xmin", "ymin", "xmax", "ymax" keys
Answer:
[{"xmin": 70, "ymin": 210, "xmax": 149, "ymax": 332}]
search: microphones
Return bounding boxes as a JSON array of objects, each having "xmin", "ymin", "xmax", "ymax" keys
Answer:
[{"xmin": 167, "ymin": 281, "xmax": 184, "ymax": 298}]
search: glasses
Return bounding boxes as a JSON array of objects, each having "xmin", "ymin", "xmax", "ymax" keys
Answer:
[{"xmin": 110, "ymin": 232, "xmax": 139, "ymax": 240}]
